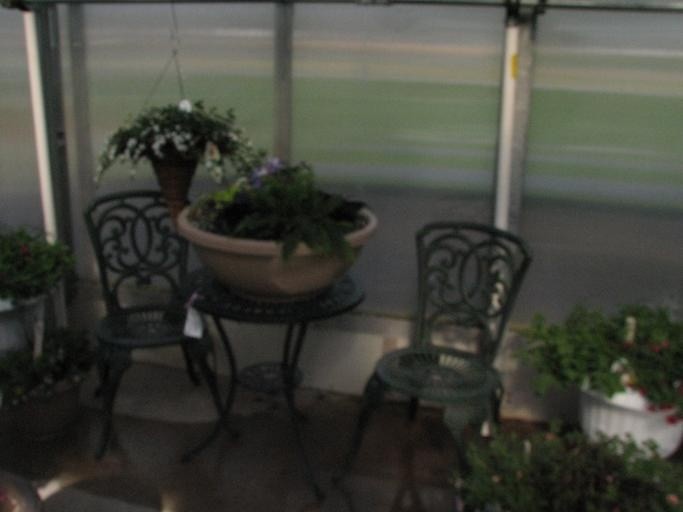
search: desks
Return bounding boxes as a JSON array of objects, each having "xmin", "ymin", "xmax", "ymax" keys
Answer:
[{"xmin": 179, "ymin": 264, "xmax": 366, "ymax": 502}]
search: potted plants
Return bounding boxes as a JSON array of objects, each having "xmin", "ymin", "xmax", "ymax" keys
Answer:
[{"xmin": 0, "ymin": 325, "xmax": 94, "ymax": 449}]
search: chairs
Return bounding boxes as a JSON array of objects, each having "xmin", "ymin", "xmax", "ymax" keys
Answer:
[
  {"xmin": 330, "ymin": 218, "xmax": 533, "ymax": 511},
  {"xmin": 81, "ymin": 189, "xmax": 242, "ymax": 464}
]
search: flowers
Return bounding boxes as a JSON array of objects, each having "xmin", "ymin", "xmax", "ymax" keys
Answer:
[
  {"xmin": 450, "ymin": 413, "xmax": 683, "ymax": 511},
  {"xmin": 93, "ymin": 99, "xmax": 266, "ymax": 187},
  {"xmin": 0, "ymin": 222, "xmax": 82, "ymax": 305},
  {"xmin": 505, "ymin": 300, "xmax": 683, "ymax": 426},
  {"xmin": 187, "ymin": 157, "xmax": 374, "ymax": 265}
]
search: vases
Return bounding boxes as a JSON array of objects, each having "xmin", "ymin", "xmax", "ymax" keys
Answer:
[
  {"xmin": 577, "ymin": 382, "xmax": 683, "ymax": 462},
  {"xmin": 146, "ymin": 143, "xmax": 199, "ymax": 225},
  {"xmin": 1, "ymin": 295, "xmax": 49, "ymax": 352},
  {"xmin": 176, "ymin": 195, "xmax": 378, "ymax": 306}
]
